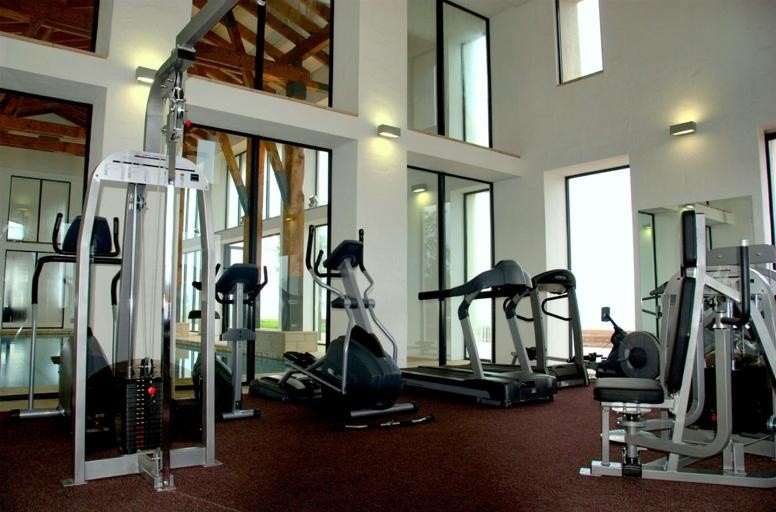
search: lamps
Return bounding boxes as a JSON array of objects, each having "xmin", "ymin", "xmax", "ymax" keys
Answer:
[
  {"xmin": 410, "ymin": 183, "xmax": 427, "ymax": 194},
  {"xmin": 135, "ymin": 65, "xmax": 160, "ymax": 85},
  {"xmin": 669, "ymin": 119, "xmax": 697, "ymax": 137},
  {"xmin": 375, "ymin": 123, "xmax": 402, "ymax": 140}
]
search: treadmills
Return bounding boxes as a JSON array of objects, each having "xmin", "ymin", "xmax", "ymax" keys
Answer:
[{"xmin": 399, "ymin": 260, "xmax": 590, "ymax": 409}]
